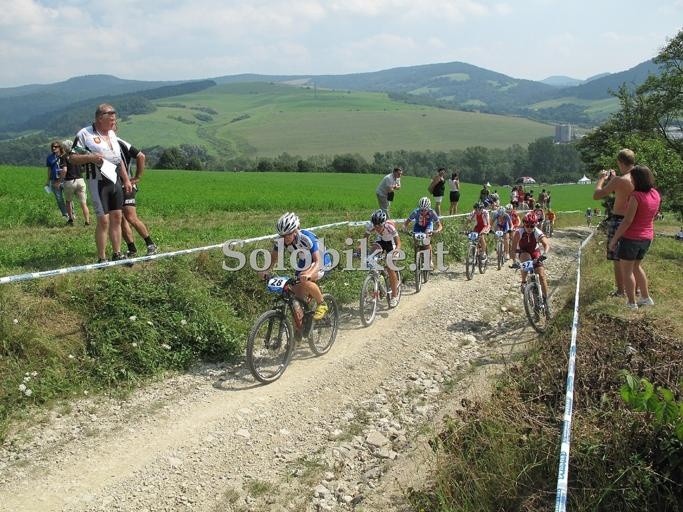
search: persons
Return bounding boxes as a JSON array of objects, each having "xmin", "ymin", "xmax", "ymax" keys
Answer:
[
  {"xmin": 429, "ymin": 167, "xmax": 446, "ymax": 219},
  {"xmin": 449, "ymin": 172, "xmax": 461, "ymax": 215},
  {"xmin": 401, "ymin": 197, "xmax": 443, "ymax": 274},
  {"xmin": 594, "ymin": 208, "xmax": 601, "ymax": 216},
  {"xmin": 595, "ymin": 149, "xmax": 641, "ymax": 296},
  {"xmin": 655, "ymin": 212, "xmax": 665, "ymax": 221},
  {"xmin": 376, "ymin": 167, "xmax": 402, "ymax": 220},
  {"xmin": 585, "ymin": 208, "xmax": 593, "ymax": 223},
  {"xmin": 45, "ymin": 103, "xmax": 158, "ymax": 264},
  {"xmin": 257, "ymin": 211, "xmax": 329, "ymax": 340},
  {"xmin": 462, "ymin": 185, "xmax": 556, "ymax": 320},
  {"xmin": 356, "ymin": 209, "xmax": 401, "ymax": 308},
  {"xmin": 677, "ymin": 227, "xmax": 683, "ymax": 240},
  {"xmin": 609, "ymin": 164, "xmax": 661, "ymax": 311}
]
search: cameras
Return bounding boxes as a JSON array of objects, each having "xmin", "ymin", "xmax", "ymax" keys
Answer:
[{"xmin": 605, "ymin": 172, "xmax": 612, "ymax": 177}]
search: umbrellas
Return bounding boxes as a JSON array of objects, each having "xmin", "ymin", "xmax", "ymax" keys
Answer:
[{"xmin": 516, "ymin": 176, "xmax": 537, "ymax": 193}]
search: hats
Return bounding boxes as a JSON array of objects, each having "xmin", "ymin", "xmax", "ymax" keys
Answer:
[{"xmin": 63, "ymin": 140, "xmax": 74, "ymax": 153}]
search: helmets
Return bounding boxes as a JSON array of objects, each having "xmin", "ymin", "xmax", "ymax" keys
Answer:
[
  {"xmin": 419, "ymin": 196, "xmax": 432, "ymax": 210},
  {"xmin": 275, "ymin": 212, "xmax": 302, "ymax": 237},
  {"xmin": 473, "ymin": 200, "xmax": 513, "ymax": 216},
  {"xmin": 522, "ymin": 212, "xmax": 538, "ymax": 223},
  {"xmin": 371, "ymin": 209, "xmax": 387, "ymax": 225}
]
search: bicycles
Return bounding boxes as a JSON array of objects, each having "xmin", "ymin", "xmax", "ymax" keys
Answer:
[
  {"xmin": 508, "ymin": 258, "xmax": 551, "ymax": 332},
  {"xmin": 399, "ymin": 228, "xmax": 434, "ymax": 292},
  {"xmin": 543, "ymin": 220, "xmax": 554, "ymax": 238},
  {"xmin": 587, "ymin": 215, "xmax": 592, "ymax": 227},
  {"xmin": 490, "ymin": 227, "xmax": 521, "ymax": 270},
  {"xmin": 458, "ymin": 231, "xmax": 489, "ymax": 280},
  {"xmin": 245, "ymin": 274, "xmax": 338, "ymax": 383},
  {"xmin": 351, "ymin": 252, "xmax": 402, "ymax": 327}
]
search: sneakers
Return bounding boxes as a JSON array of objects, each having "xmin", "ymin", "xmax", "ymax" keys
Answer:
[
  {"xmin": 85, "ymin": 221, "xmax": 91, "ymax": 226},
  {"xmin": 610, "ymin": 289, "xmax": 654, "ymax": 310},
  {"xmin": 313, "ymin": 300, "xmax": 329, "ymax": 320},
  {"xmin": 95, "ymin": 243, "xmax": 160, "ymax": 269},
  {"xmin": 66, "ymin": 220, "xmax": 73, "ymax": 226},
  {"xmin": 521, "ymin": 282, "xmax": 528, "ymax": 293},
  {"xmin": 545, "ymin": 306, "xmax": 552, "ymax": 319},
  {"xmin": 389, "ymin": 292, "xmax": 400, "ymax": 307},
  {"xmin": 480, "ymin": 252, "xmax": 488, "ymax": 263}
]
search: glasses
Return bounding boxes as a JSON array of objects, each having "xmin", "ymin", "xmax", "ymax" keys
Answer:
[
  {"xmin": 53, "ymin": 146, "xmax": 59, "ymax": 148},
  {"xmin": 104, "ymin": 110, "xmax": 116, "ymax": 115}
]
